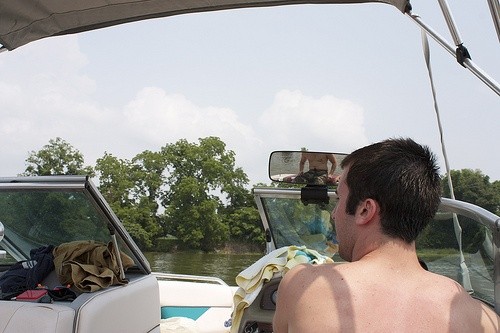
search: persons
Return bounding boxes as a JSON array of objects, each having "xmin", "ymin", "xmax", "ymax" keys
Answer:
[
  {"xmin": 294, "ymin": 152, "xmax": 337, "ymax": 183},
  {"xmin": 272, "ymin": 139, "xmax": 500, "ymax": 333}
]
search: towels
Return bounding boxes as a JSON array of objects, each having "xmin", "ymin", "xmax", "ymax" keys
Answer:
[{"xmin": 229, "ymin": 245, "xmax": 335, "ymax": 333}]
